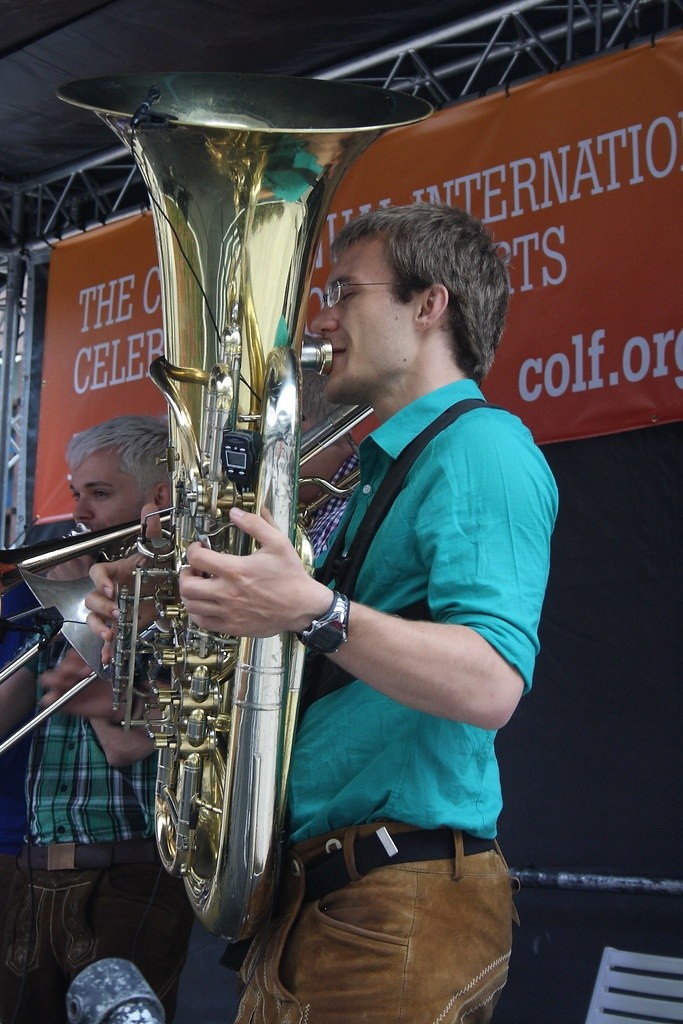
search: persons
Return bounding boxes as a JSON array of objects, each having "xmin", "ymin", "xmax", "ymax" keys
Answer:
[
  {"xmin": 85, "ymin": 198, "xmax": 560, "ymax": 1024},
  {"xmin": 0, "ymin": 373, "xmax": 372, "ymax": 1024}
]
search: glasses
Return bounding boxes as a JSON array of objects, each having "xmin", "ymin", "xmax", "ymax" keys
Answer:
[{"xmin": 321, "ymin": 279, "xmax": 428, "ymax": 308}]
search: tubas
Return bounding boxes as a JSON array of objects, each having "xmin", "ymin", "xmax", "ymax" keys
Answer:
[{"xmin": 55, "ymin": 72, "xmax": 438, "ymax": 945}]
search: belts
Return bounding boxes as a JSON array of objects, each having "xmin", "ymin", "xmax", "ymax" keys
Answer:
[
  {"xmin": 219, "ymin": 826, "xmax": 496, "ymax": 972},
  {"xmin": 20, "ymin": 836, "xmax": 162, "ymax": 870}
]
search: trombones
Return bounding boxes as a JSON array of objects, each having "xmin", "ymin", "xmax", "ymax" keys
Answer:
[{"xmin": 0, "ymin": 396, "xmax": 377, "ymax": 754}]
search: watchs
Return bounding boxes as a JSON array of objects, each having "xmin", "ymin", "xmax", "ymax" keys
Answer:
[{"xmin": 295, "ymin": 590, "xmax": 353, "ymax": 657}]
